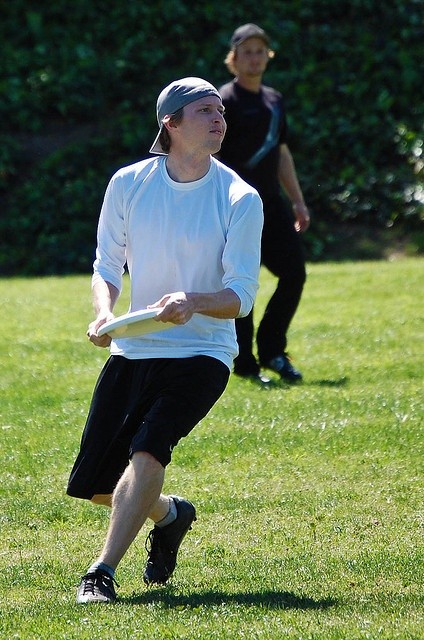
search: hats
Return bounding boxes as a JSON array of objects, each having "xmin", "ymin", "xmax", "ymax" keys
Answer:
[
  {"xmin": 232, "ymin": 24, "xmax": 269, "ymax": 47},
  {"xmin": 148, "ymin": 77, "xmax": 222, "ymax": 155}
]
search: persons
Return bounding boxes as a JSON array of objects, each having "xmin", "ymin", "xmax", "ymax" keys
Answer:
[
  {"xmin": 216, "ymin": 22, "xmax": 309, "ymax": 386},
  {"xmin": 66, "ymin": 76, "xmax": 265, "ymax": 607}
]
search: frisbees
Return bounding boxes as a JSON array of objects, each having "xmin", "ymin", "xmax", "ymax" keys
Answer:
[{"xmin": 96, "ymin": 306, "xmax": 179, "ymax": 339}]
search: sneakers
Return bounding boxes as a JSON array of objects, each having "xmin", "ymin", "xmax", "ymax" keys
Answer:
[
  {"xmin": 143, "ymin": 495, "xmax": 196, "ymax": 585},
  {"xmin": 265, "ymin": 352, "xmax": 302, "ymax": 381},
  {"xmin": 75, "ymin": 568, "xmax": 117, "ymax": 605},
  {"xmin": 234, "ymin": 354, "xmax": 261, "ymax": 379}
]
